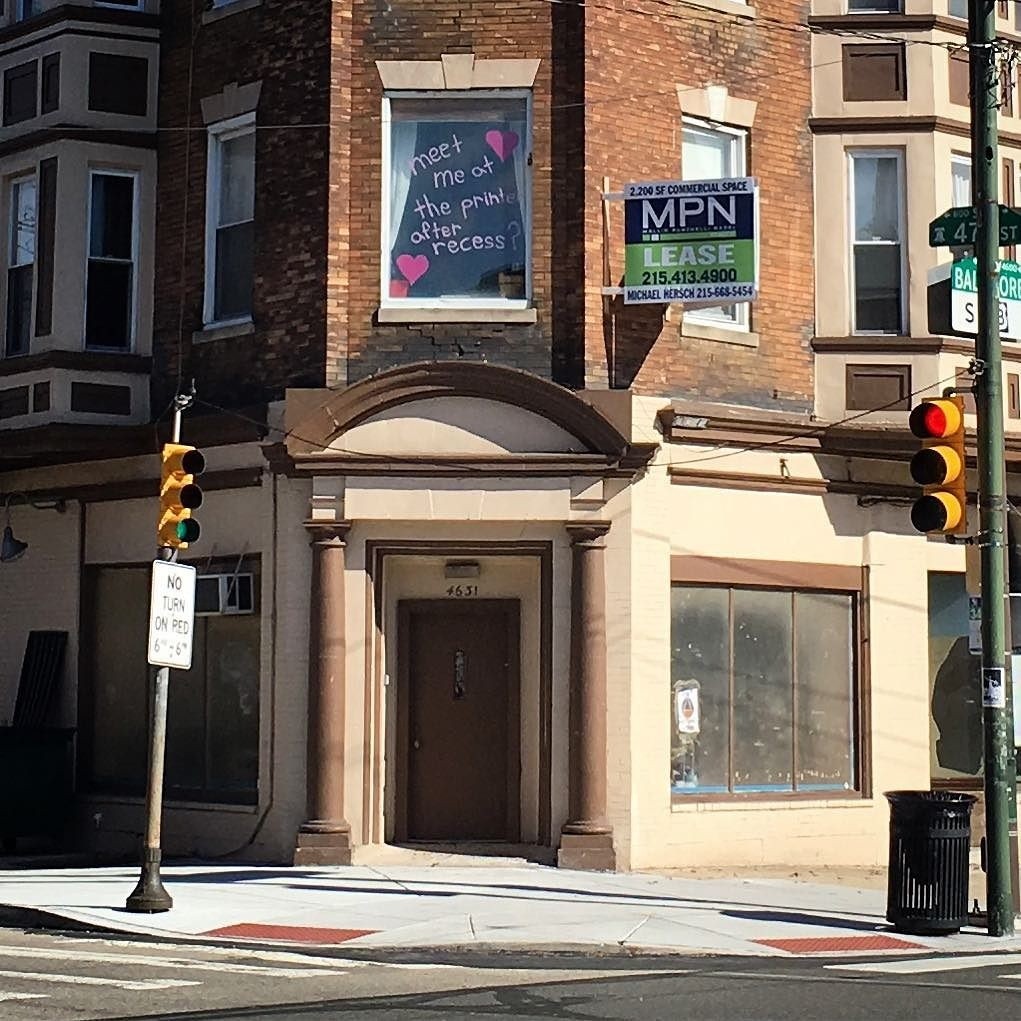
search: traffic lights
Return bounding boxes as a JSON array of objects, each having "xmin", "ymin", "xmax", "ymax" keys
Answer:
[
  {"xmin": 907, "ymin": 396, "xmax": 967, "ymax": 536},
  {"xmin": 156, "ymin": 443, "xmax": 206, "ymax": 548}
]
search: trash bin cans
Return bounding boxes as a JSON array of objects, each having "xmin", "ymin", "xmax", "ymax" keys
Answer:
[{"xmin": 881, "ymin": 789, "xmax": 980, "ymax": 934}]
